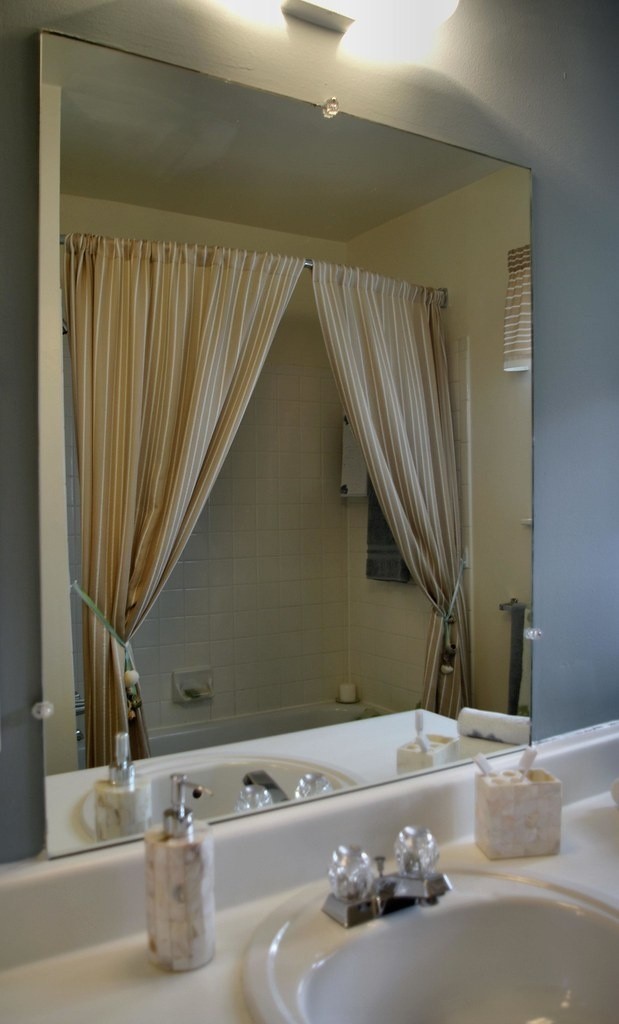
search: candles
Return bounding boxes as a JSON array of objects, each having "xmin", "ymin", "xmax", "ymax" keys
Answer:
[{"xmin": 338, "ymin": 682, "xmax": 356, "ymax": 702}]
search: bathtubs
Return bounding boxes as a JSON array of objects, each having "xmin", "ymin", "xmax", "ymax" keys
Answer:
[{"xmin": 143, "ymin": 700, "xmax": 398, "ymax": 758}]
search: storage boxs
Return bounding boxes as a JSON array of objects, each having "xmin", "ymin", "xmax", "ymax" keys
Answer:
[
  {"xmin": 396, "ymin": 733, "xmax": 461, "ymax": 777},
  {"xmin": 473, "ymin": 766, "xmax": 562, "ymax": 864}
]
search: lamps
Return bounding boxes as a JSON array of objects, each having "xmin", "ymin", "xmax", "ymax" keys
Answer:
[{"xmin": 281, "ymin": 0, "xmax": 355, "ymax": 34}]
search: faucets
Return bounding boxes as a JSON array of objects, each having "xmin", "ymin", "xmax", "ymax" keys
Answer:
[
  {"xmin": 319, "ymin": 825, "xmax": 455, "ymax": 930},
  {"xmin": 233, "ymin": 768, "xmax": 332, "ymax": 814}
]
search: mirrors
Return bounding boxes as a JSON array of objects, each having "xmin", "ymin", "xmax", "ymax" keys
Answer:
[{"xmin": 35, "ymin": 23, "xmax": 538, "ymax": 855}]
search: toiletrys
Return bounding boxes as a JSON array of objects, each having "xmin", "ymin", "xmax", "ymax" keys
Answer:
[
  {"xmin": 91, "ymin": 729, "xmax": 147, "ymax": 845},
  {"xmin": 142, "ymin": 771, "xmax": 219, "ymax": 975}
]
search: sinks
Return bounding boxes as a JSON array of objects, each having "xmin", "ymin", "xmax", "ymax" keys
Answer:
[
  {"xmin": 241, "ymin": 866, "xmax": 619, "ymax": 1024},
  {"xmin": 79, "ymin": 754, "xmax": 373, "ymax": 846}
]
